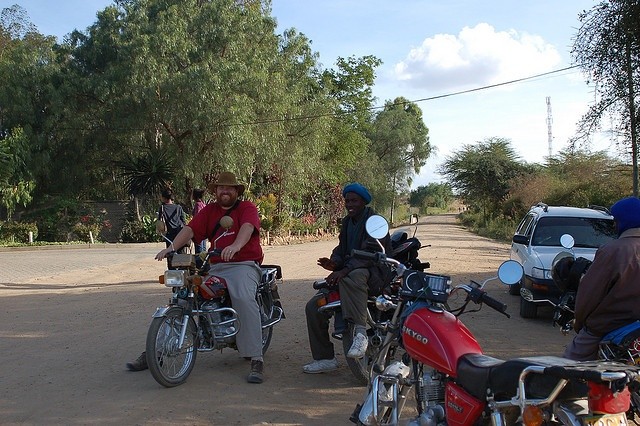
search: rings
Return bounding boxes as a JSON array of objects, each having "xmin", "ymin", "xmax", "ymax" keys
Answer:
[{"xmin": 229, "ymin": 250, "xmax": 232, "ymax": 254}]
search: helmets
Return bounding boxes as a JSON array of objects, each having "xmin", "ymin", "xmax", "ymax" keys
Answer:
[{"xmin": 552, "ymin": 252, "xmax": 577, "ymax": 268}]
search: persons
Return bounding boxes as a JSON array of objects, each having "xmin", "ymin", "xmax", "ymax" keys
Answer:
[
  {"xmin": 191, "ymin": 188, "xmax": 207, "ymax": 254},
  {"xmin": 158, "ymin": 190, "xmax": 190, "ymax": 271},
  {"xmin": 562, "ymin": 196, "xmax": 640, "ymax": 363},
  {"xmin": 124, "ymin": 171, "xmax": 266, "ymax": 383},
  {"xmin": 302, "ymin": 183, "xmax": 391, "ymax": 375}
]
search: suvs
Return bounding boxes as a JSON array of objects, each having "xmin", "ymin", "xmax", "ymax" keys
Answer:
[{"xmin": 510, "ymin": 202, "xmax": 617, "ymax": 318}]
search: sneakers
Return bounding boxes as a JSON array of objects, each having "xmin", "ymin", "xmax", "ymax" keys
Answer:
[
  {"xmin": 347, "ymin": 333, "xmax": 369, "ymax": 359},
  {"xmin": 126, "ymin": 351, "xmax": 163, "ymax": 371},
  {"xmin": 247, "ymin": 360, "xmax": 265, "ymax": 384},
  {"xmin": 302, "ymin": 359, "xmax": 338, "ymax": 374}
]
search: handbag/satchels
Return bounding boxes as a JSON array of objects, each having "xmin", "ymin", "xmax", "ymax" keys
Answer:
[
  {"xmin": 156, "ymin": 204, "xmax": 168, "ymax": 236},
  {"xmin": 208, "ymin": 199, "xmax": 242, "ymax": 242}
]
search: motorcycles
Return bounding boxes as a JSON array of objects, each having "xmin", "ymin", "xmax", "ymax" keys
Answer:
[
  {"xmin": 350, "ymin": 215, "xmax": 640, "ymax": 426},
  {"xmin": 315, "ymin": 214, "xmax": 431, "ymax": 385},
  {"xmin": 520, "ymin": 234, "xmax": 640, "ymax": 366},
  {"xmin": 145, "ymin": 215, "xmax": 285, "ymax": 387}
]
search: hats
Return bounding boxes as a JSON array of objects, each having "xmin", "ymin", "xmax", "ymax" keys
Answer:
[
  {"xmin": 208, "ymin": 171, "xmax": 245, "ymax": 197},
  {"xmin": 343, "ymin": 182, "xmax": 371, "ymax": 202},
  {"xmin": 610, "ymin": 196, "xmax": 640, "ymax": 233}
]
